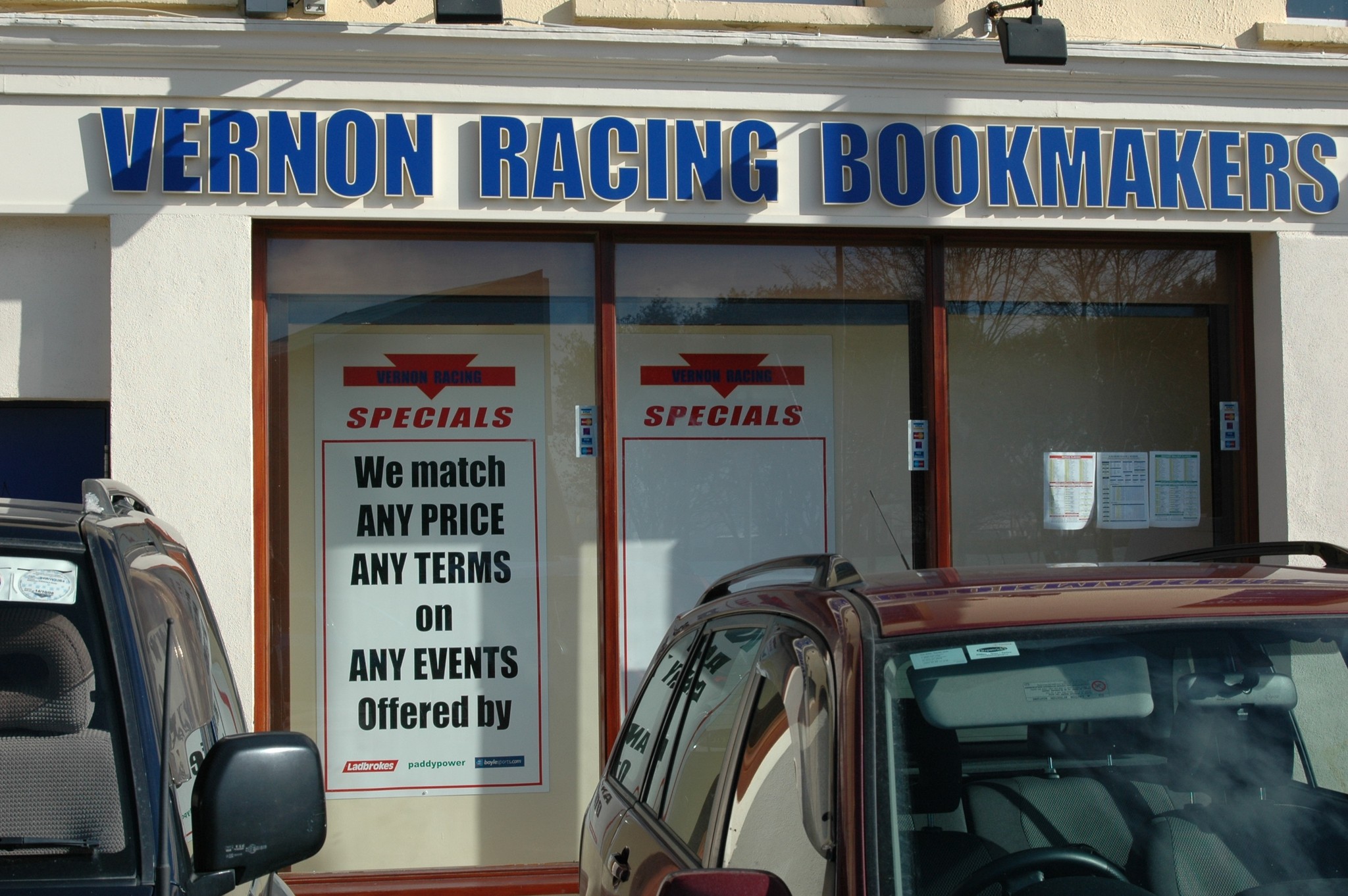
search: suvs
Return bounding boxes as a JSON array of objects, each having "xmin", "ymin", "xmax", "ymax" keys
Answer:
[
  {"xmin": 577, "ymin": 540, "xmax": 1348, "ymax": 896},
  {"xmin": 1, "ymin": 477, "xmax": 328, "ymax": 896}
]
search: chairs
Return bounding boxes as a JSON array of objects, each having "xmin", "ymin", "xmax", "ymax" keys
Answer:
[
  {"xmin": 1122, "ymin": 690, "xmax": 1348, "ymax": 896},
  {"xmin": 0, "ymin": 606, "xmax": 126, "ymax": 855},
  {"xmin": 892, "ymin": 697, "xmax": 1034, "ymax": 896}
]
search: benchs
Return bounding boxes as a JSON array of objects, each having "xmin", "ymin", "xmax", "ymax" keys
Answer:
[{"xmin": 896, "ymin": 721, "xmax": 1206, "ymax": 866}]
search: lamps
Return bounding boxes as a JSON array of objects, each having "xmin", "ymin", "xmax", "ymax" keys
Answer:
[
  {"xmin": 433, "ymin": 0, "xmax": 504, "ymax": 24},
  {"xmin": 985, "ymin": 0, "xmax": 1068, "ymax": 67}
]
